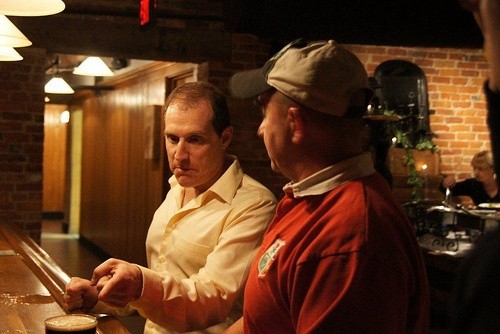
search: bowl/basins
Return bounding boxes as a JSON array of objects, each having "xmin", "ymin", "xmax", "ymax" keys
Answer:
[{"xmin": 455, "ymin": 196, "xmax": 476, "ymax": 209}]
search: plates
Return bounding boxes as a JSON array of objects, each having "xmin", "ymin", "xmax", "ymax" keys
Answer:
[{"xmin": 478, "ymin": 203, "xmax": 500, "ymax": 209}]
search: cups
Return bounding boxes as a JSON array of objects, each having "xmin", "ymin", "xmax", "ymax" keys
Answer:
[{"xmin": 44, "ymin": 314, "xmax": 98, "ymax": 334}]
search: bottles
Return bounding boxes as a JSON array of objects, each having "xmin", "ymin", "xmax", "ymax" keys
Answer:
[{"xmin": 441, "ymin": 186, "xmax": 458, "ymax": 227}]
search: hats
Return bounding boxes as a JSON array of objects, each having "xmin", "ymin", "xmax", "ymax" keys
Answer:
[{"xmin": 230, "ymin": 38, "xmax": 375, "ymax": 119}]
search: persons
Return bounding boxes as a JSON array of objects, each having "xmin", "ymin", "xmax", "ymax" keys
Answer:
[
  {"xmin": 63, "ymin": 81, "xmax": 279, "ymax": 334},
  {"xmin": 439, "ymin": 151, "xmax": 500, "ymax": 217},
  {"xmin": 221, "ymin": 40, "xmax": 432, "ymax": 334}
]
